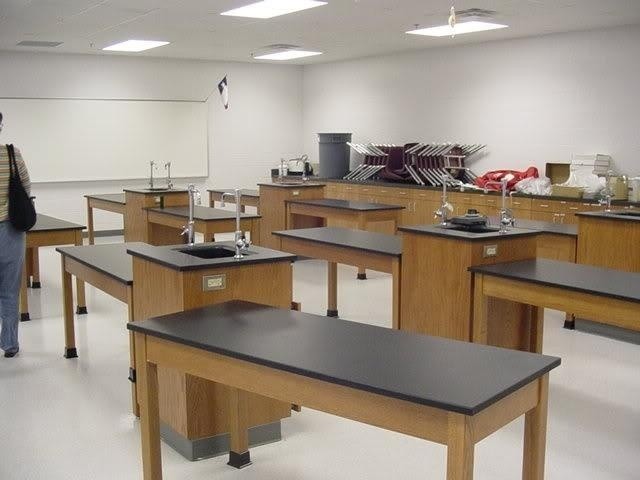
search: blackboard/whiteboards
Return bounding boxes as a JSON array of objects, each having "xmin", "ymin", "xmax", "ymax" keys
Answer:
[{"xmin": 0, "ymin": 96, "xmax": 208, "ymax": 183}]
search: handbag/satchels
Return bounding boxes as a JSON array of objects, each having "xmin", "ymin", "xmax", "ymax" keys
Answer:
[{"xmin": 7, "ymin": 144, "xmax": 36, "ymax": 232}]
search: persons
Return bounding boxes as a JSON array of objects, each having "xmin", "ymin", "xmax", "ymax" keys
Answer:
[{"xmin": 0, "ymin": 113, "xmax": 36, "ymax": 357}]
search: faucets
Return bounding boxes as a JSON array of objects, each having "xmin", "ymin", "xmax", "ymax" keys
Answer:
[
  {"xmin": 220, "ymin": 189, "xmax": 252, "ymax": 258},
  {"xmin": 483, "ymin": 179, "xmax": 515, "ymax": 234},
  {"xmin": 599, "ymin": 170, "xmax": 615, "ymax": 212},
  {"xmin": 164, "ymin": 161, "xmax": 173, "ymax": 189},
  {"xmin": 289, "ymin": 154, "xmax": 312, "ymax": 182},
  {"xmin": 278, "ymin": 157, "xmax": 287, "ymax": 182},
  {"xmin": 181, "ymin": 184, "xmax": 201, "ymax": 244},
  {"xmin": 434, "ymin": 176, "xmax": 464, "ymax": 227},
  {"xmin": 147, "ymin": 160, "xmax": 158, "ymax": 187}
]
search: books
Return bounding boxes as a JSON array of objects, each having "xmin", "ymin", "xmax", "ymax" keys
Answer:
[{"xmin": 571, "ymin": 154, "xmax": 611, "ymax": 175}]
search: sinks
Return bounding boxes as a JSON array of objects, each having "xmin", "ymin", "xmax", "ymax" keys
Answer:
[
  {"xmin": 610, "ymin": 211, "xmax": 640, "ymax": 217},
  {"xmin": 280, "ymin": 182, "xmax": 302, "ymax": 185},
  {"xmin": 173, "ymin": 245, "xmax": 258, "ymax": 258},
  {"xmin": 145, "ymin": 188, "xmax": 168, "ymax": 191},
  {"xmin": 445, "ymin": 225, "xmax": 509, "ymax": 233}
]
